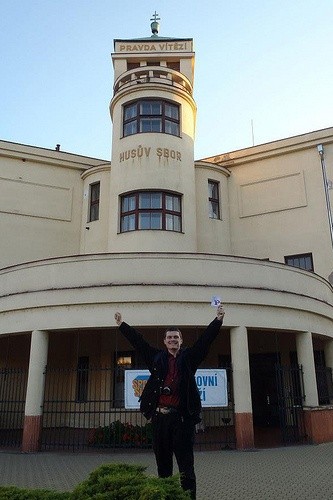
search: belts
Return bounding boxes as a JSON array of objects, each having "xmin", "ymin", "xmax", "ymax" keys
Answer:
[{"xmin": 153, "ymin": 405, "xmax": 179, "ymax": 416}]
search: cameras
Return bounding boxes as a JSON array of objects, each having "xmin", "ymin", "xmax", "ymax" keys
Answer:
[{"xmin": 157, "ymin": 384, "xmax": 173, "ymax": 396}]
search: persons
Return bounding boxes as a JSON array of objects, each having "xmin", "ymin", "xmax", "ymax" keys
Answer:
[{"xmin": 113, "ymin": 305, "xmax": 225, "ymax": 500}]
